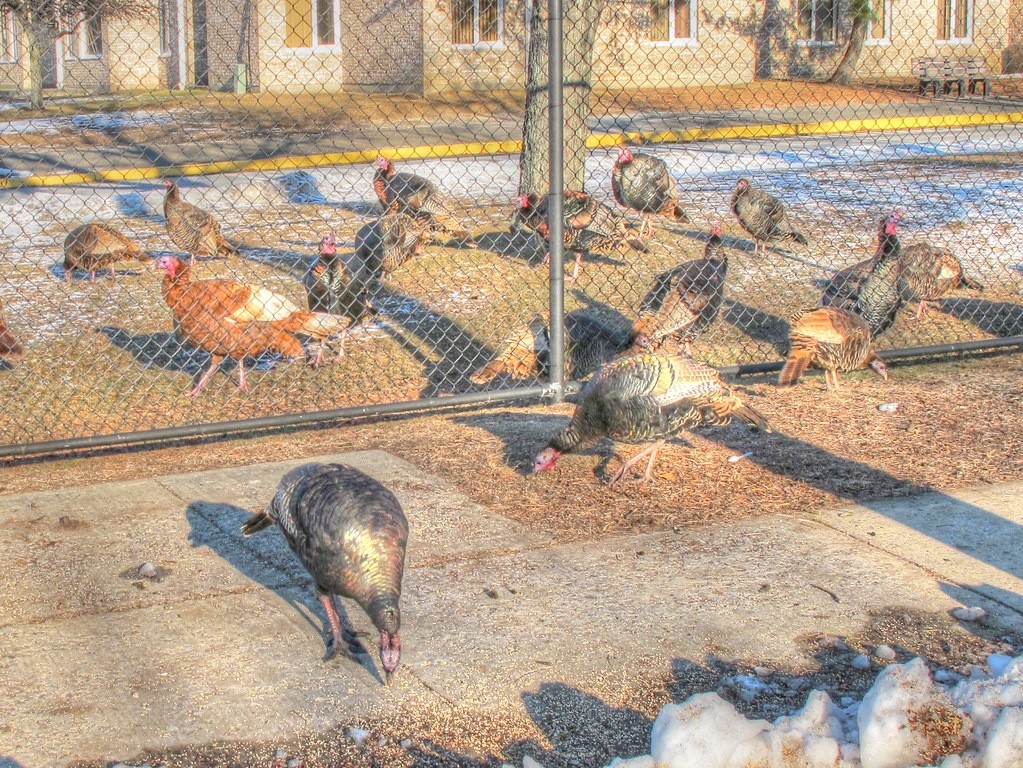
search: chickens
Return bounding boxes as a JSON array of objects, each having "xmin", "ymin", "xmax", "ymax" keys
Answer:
[
  {"xmin": 611, "ymin": 148, "xmax": 691, "ymax": 240},
  {"xmin": 0, "ymin": 299, "xmax": 23, "ymax": 366},
  {"xmin": 776, "ymin": 305, "xmax": 888, "ymax": 396},
  {"xmin": 637, "ymin": 227, "xmax": 729, "ymax": 358},
  {"xmin": 531, "ymin": 354, "xmax": 772, "ymax": 494},
  {"xmin": 158, "ymin": 179, "xmax": 241, "ymax": 268},
  {"xmin": 149, "ymin": 255, "xmax": 352, "ymax": 401},
  {"xmin": 820, "ymin": 207, "xmax": 986, "ymax": 351},
  {"xmin": 562, "ymin": 303, "xmax": 655, "ymax": 381},
  {"xmin": 514, "ymin": 189, "xmax": 653, "ymax": 284},
  {"xmin": 372, "ymin": 155, "xmax": 478, "ymax": 249},
  {"xmin": 62, "ymin": 223, "xmax": 154, "ymax": 291},
  {"xmin": 730, "ymin": 179, "xmax": 809, "ymax": 260},
  {"xmin": 354, "ymin": 210, "xmax": 426, "ymax": 287},
  {"xmin": 240, "ymin": 461, "xmax": 411, "ymax": 687},
  {"xmin": 304, "ymin": 229, "xmax": 370, "ymax": 370},
  {"xmin": 469, "ymin": 312, "xmax": 550, "ymax": 385}
]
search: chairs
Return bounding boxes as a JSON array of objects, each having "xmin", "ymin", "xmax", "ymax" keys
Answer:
[{"xmin": 913, "ymin": 55, "xmax": 992, "ymax": 100}]
